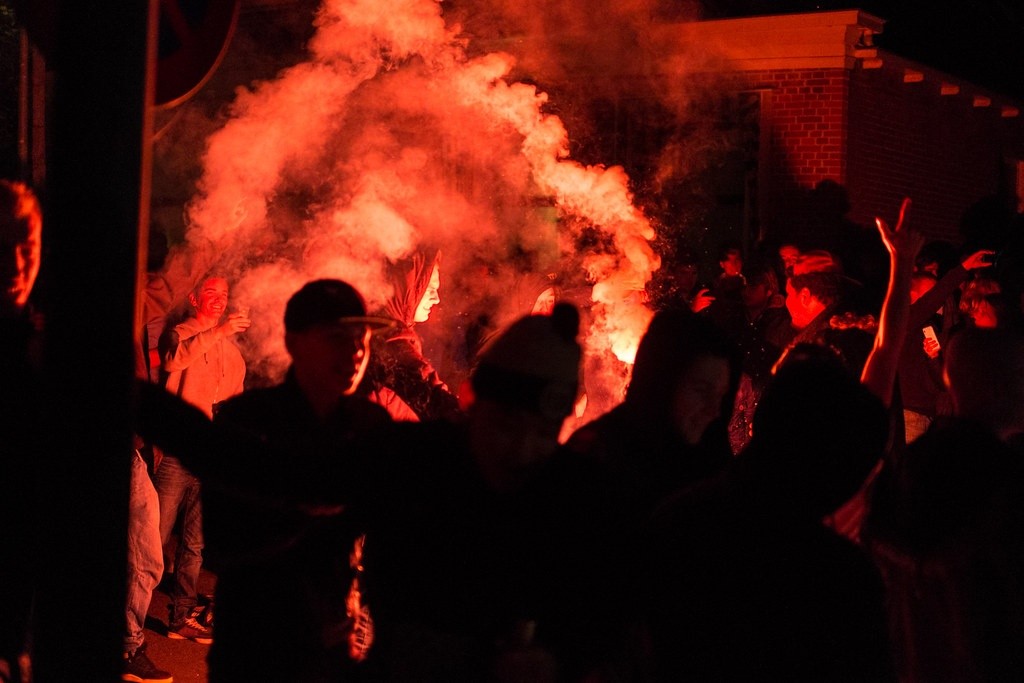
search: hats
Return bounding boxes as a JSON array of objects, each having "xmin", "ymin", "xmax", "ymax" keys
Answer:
[
  {"xmin": 285, "ymin": 279, "xmax": 390, "ymax": 332},
  {"xmin": 481, "ymin": 302, "xmax": 581, "ymax": 387}
]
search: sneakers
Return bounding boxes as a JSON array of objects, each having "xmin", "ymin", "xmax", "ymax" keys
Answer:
[
  {"xmin": 167, "ymin": 617, "xmax": 215, "ymax": 644},
  {"xmin": 121, "ymin": 649, "xmax": 172, "ymax": 683}
]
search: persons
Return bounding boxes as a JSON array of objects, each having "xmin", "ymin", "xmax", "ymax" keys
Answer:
[{"xmin": 0, "ymin": 182, "xmax": 1024, "ymax": 683}]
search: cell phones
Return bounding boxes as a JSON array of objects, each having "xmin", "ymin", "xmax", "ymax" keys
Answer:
[
  {"xmin": 238, "ymin": 306, "xmax": 249, "ymax": 317},
  {"xmin": 923, "ymin": 326, "xmax": 940, "ymax": 350},
  {"xmin": 702, "ymin": 281, "xmax": 712, "ymax": 311}
]
